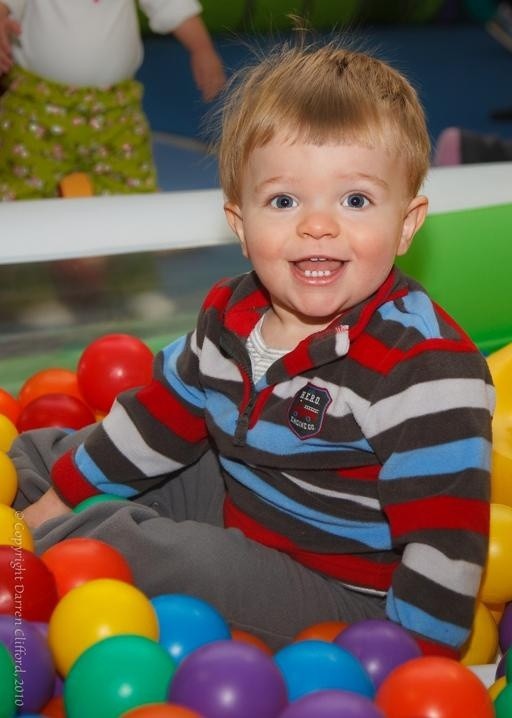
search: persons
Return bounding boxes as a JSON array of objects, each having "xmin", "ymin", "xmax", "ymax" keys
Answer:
[
  {"xmin": 0, "ymin": 0, "xmax": 226, "ymax": 200},
  {"xmin": 12, "ymin": 45, "xmax": 498, "ymax": 661},
  {"xmin": 0, "ymin": 331, "xmax": 512, "ymax": 718}
]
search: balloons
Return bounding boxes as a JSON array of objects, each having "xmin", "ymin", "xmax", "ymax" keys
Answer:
[{"xmin": 0, "ymin": 331, "xmax": 512, "ymax": 718}]
